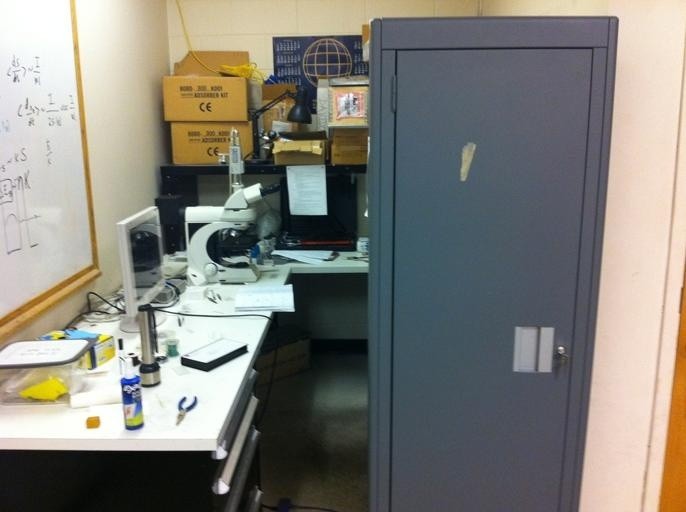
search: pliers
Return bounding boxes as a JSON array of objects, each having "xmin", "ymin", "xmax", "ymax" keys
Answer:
[{"xmin": 176, "ymin": 397, "xmax": 196, "ymax": 425}]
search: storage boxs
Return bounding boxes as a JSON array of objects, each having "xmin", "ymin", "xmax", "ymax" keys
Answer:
[
  {"xmin": 162, "ymin": 50, "xmax": 256, "ymax": 166},
  {"xmin": 261, "ymin": 83, "xmax": 299, "ymax": 133},
  {"xmin": 330, "ymin": 127, "xmax": 368, "ymax": 164},
  {"xmin": 273, "ymin": 130, "xmax": 328, "ymax": 166}
]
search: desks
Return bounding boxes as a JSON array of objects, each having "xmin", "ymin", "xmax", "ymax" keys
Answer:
[
  {"xmin": 0, "ymin": 266, "xmax": 292, "ymax": 512},
  {"xmin": 164, "ymin": 252, "xmax": 368, "ymax": 274}
]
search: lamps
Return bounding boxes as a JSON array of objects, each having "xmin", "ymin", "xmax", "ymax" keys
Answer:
[{"xmin": 246, "ymin": 85, "xmax": 312, "ymax": 166}]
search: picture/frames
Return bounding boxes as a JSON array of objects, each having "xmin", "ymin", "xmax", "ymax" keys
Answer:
[{"xmin": 316, "ymin": 77, "xmax": 369, "ymax": 130}]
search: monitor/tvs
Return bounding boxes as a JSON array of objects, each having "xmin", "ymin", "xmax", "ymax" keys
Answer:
[{"xmin": 117, "ymin": 206, "xmax": 166, "ymax": 332}]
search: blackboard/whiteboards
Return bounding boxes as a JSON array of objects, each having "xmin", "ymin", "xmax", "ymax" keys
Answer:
[{"xmin": 1, "ymin": 1, "xmax": 101, "ymax": 341}]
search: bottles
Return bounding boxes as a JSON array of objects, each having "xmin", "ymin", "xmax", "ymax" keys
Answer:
[{"xmin": 120, "ymin": 356, "xmax": 145, "ymax": 430}]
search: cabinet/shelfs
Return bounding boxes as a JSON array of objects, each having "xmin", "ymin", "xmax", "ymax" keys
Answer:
[{"xmin": 370, "ymin": 17, "xmax": 620, "ymax": 510}]
